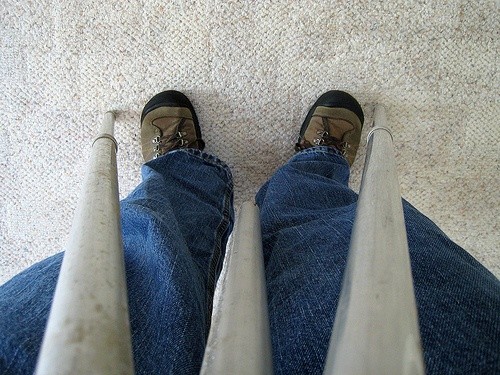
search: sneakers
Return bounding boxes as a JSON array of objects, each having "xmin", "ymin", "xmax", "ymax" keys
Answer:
[
  {"xmin": 141, "ymin": 90, "xmax": 205, "ymax": 162},
  {"xmin": 293, "ymin": 90, "xmax": 365, "ymax": 167}
]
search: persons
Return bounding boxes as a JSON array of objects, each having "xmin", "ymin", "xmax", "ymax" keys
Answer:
[{"xmin": 0, "ymin": 87, "xmax": 500, "ymax": 375}]
